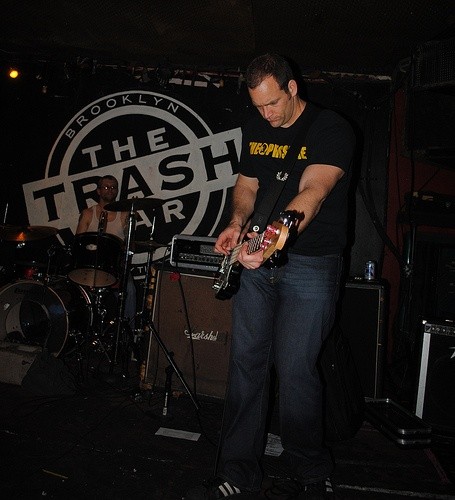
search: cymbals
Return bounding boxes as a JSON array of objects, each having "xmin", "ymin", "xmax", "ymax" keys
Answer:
[
  {"xmin": 103, "ymin": 198, "xmax": 161, "ymax": 212},
  {"xmin": 135, "ymin": 241, "xmax": 167, "ymax": 247},
  {"xmin": 0, "ymin": 226, "xmax": 58, "ymax": 241}
]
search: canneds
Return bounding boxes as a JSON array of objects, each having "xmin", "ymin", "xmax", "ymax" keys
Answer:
[{"xmin": 364, "ymin": 261, "xmax": 375, "ymax": 280}]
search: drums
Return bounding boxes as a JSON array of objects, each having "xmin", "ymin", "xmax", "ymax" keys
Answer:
[
  {"xmin": 0, "ymin": 247, "xmax": 56, "ymax": 288},
  {"xmin": 0, "ymin": 275, "xmax": 93, "ymax": 358},
  {"xmin": 66, "ymin": 232, "xmax": 125, "ymax": 287}
]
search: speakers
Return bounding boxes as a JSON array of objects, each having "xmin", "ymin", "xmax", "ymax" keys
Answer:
[{"xmin": 144, "ymin": 271, "xmax": 232, "ymax": 400}]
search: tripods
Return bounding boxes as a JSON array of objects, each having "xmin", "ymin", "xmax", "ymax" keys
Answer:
[{"xmin": 95, "ymin": 206, "xmax": 141, "ymax": 366}]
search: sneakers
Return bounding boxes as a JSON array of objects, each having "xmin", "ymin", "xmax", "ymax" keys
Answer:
[
  {"xmin": 303, "ymin": 477, "xmax": 334, "ymax": 500},
  {"xmin": 180, "ymin": 477, "xmax": 260, "ymax": 500}
]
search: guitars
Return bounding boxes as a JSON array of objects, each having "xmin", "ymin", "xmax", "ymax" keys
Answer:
[{"xmin": 210, "ymin": 209, "xmax": 299, "ymax": 301}]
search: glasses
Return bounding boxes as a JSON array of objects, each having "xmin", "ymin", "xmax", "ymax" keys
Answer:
[{"xmin": 100, "ymin": 185, "xmax": 117, "ymax": 190}]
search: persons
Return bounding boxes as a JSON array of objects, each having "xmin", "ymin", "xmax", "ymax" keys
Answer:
[
  {"xmin": 211, "ymin": 53, "xmax": 355, "ymax": 500},
  {"xmin": 76, "ymin": 175, "xmax": 136, "ymax": 329}
]
